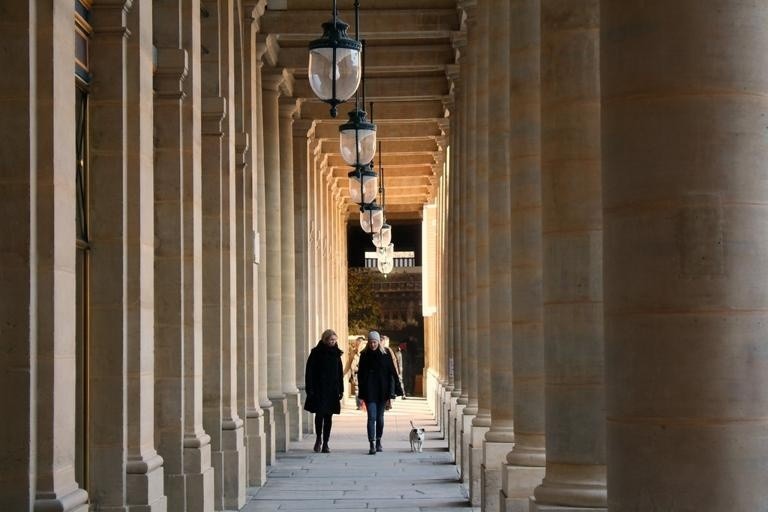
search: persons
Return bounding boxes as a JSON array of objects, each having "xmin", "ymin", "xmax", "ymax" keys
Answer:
[
  {"xmin": 350, "ymin": 331, "xmax": 408, "ymax": 455},
  {"xmin": 303, "ymin": 329, "xmax": 345, "ymax": 453}
]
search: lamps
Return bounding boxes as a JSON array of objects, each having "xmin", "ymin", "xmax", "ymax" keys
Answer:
[{"xmin": 307, "ymin": 0, "xmax": 395, "ymax": 280}]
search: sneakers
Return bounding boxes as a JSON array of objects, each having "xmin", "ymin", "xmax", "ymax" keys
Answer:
[
  {"xmin": 322, "ymin": 444, "xmax": 329, "ymax": 453},
  {"xmin": 314, "ymin": 440, "xmax": 322, "ymax": 452}
]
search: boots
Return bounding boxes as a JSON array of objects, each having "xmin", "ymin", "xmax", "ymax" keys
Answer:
[
  {"xmin": 369, "ymin": 441, "xmax": 376, "ymax": 454},
  {"xmin": 376, "ymin": 439, "xmax": 382, "ymax": 452}
]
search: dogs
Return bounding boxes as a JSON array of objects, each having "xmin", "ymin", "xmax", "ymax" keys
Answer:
[{"xmin": 410, "ymin": 421, "xmax": 425, "ymax": 453}]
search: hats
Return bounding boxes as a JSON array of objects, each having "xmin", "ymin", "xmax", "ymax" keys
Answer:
[{"xmin": 368, "ymin": 331, "xmax": 380, "ymax": 343}]
